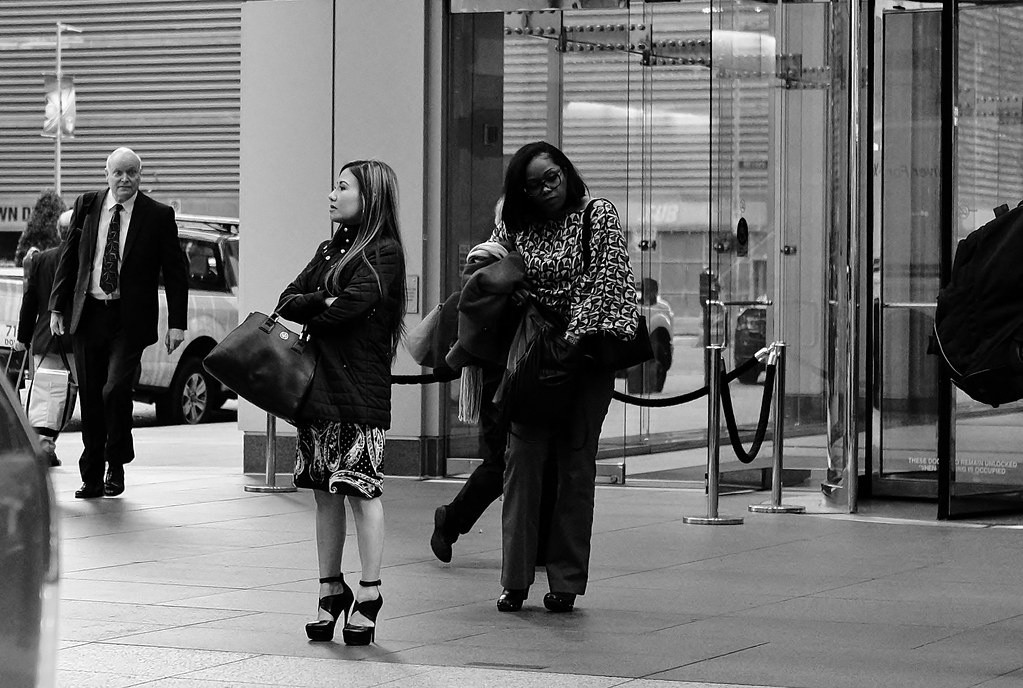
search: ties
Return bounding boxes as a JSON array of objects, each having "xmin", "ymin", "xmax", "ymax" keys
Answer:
[{"xmin": 99, "ymin": 204, "xmax": 123, "ymax": 295}]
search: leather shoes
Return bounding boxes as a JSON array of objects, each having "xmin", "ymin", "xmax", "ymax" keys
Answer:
[
  {"xmin": 75, "ymin": 481, "xmax": 104, "ymax": 497},
  {"xmin": 105, "ymin": 463, "xmax": 124, "ymax": 496},
  {"xmin": 543, "ymin": 591, "xmax": 575, "ymax": 611},
  {"xmin": 497, "ymin": 588, "xmax": 528, "ymax": 611}
]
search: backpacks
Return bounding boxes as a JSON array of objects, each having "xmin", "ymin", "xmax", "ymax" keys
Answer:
[{"xmin": 931, "ymin": 202, "xmax": 1023, "ymax": 407}]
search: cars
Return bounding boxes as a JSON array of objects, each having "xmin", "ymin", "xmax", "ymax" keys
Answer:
[{"xmin": 734, "ymin": 294, "xmax": 766, "ymax": 384}]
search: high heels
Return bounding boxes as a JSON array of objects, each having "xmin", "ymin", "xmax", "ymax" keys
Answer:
[
  {"xmin": 305, "ymin": 574, "xmax": 354, "ymax": 641},
  {"xmin": 343, "ymin": 580, "xmax": 383, "ymax": 644}
]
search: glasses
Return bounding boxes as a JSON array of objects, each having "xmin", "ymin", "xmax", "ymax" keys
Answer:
[{"xmin": 521, "ymin": 167, "xmax": 562, "ymax": 197}]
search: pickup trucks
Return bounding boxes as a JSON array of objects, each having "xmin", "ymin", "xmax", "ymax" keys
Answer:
[{"xmin": 0, "ymin": 213, "xmax": 240, "ymax": 426}]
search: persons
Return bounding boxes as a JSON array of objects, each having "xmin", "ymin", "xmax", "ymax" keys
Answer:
[
  {"xmin": 15, "ymin": 189, "xmax": 66, "ymax": 267},
  {"xmin": 448, "ymin": 141, "xmax": 638, "ymax": 612},
  {"xmin": 278, "ymin": 160, "xmax": 408, "ymax": 645},
  {"xmin": 14, "ymin": 209, "xmax": 79, "ymax": 466},
  {"xmin": 48, "ymin": 145, "xmax": 190, "ymax": 498},
  {"xmin": 430, "ymin": 194, "xmax": 545, "ymax": 565}
]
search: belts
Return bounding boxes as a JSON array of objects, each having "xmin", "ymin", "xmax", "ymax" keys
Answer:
[{"xmin": 87, "ymin": 296, "xmax": 120, "ymax": 307}]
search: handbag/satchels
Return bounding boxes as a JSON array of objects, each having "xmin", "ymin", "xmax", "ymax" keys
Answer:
[
  {"xmin": 405, "ymin": 292, "xmax": 461, "ymax": 366},
  {"xmin": 202, "ymin": 292, "xmax": 316, "ymax": 418},
  {"xmin": 26, "ymin": 333, "xmax": 78, "ymax": 436},
  {"xmin": 492, "ymin": 303, "xmax": 615, "ymax": 427},
  {"xmin": 615, "ymin": 316, "xmax": 654, "ymax": 369},
  {"xmin": 432, "ymin": 292, "xmax": 461, "ymax": 382}
]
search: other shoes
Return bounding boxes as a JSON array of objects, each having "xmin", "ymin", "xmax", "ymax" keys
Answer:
[
  {"xmin": 48, "ymin": 452, "xmax": 61, "ymax": 467},
  {"xmin": 431, "ymin": 505, "xmax": 459, "ymax": 563}
]
